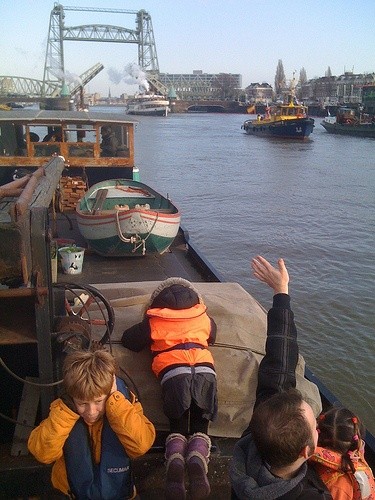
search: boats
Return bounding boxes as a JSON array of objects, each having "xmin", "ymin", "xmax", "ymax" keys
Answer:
[
  {"xmin": 127, "ymin": 88, "xmax": 171, "ymax": 117},
  {"xmin": 320, "ymin": 104, "xmax": 375, "ymax": 136},
  {"xmin": 75, "ymin": 178, "xmax": 181, "ymax": 257},
  {"xmin": 241, "ymin": 100, "xmax": 315, "ymax": 136}
]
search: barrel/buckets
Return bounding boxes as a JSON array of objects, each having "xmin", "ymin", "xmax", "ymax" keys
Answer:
[{"xmin": 57, "ymin": 247, "xmax": 85, "ymax": 275}]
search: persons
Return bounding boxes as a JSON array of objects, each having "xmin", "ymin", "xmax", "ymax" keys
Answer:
[
  {"xmin": 228, "ymin": 255, "xmax": 334, "ymax": 500},
  {"xmin": 27, "ymin": 348, "xmax": 156, "ymax": 500},
  {"xmin": 121, "ymin": 276, "xmax": 217, "ymax": 500},
  {"xmin": 101, "ymin": 126, "xmax": 118, "ymax": 156},
  {"xmin": 309, "ymin": 407, "xmax": 375, "ymax": 500}
]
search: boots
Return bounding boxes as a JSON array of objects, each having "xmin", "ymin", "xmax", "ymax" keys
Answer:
[
  {"xmin": 165, "ymin": 433, "xmax": 187, "ymax": 500},
  {"xmin": 187, "ymin": 432, "xmax": 212, "ymax": 500}
]
